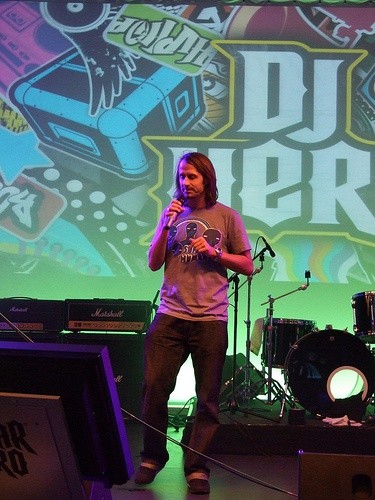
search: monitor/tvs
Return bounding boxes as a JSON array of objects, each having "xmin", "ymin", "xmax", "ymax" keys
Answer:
[{"xmin": 0, "ymin": 341, "xmax": 135, "ymax": 488}]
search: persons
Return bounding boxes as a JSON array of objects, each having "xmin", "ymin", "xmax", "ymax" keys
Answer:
[{"xmin": 133, "ymin": 152, "xmax": 254, "ymax": 494}]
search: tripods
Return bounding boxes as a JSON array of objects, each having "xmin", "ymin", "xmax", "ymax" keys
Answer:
[{"xmin": 214, "ymin": 247, "xmax": 311, "ymax": 424}]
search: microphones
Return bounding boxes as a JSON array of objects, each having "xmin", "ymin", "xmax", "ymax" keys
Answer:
[
  {"xmin": 262, "ymin": 238, "xmax": 275, "ymax": 257},
  {"xmin": 162, "ymin": 197, "xmax": 185, "ymax": 229}
]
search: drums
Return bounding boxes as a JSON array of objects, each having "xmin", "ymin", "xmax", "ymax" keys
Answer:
[
  {"xmin": 283, "ymin": 329, "xmax": 375, "ymax": 419},
  {"xmin": 351, "ymin": 290, "xmax": 375, "ymax": 345},
  {"xmin": 263, "ymin": 318, "xmax": 316, "ymax": 369}
]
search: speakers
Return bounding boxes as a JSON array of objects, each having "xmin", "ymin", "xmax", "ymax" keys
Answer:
[
  {"xmin": 219, "ymin": 352, "xmax": 269, "ymax": 403},
  {"xmin": 0, "ymin": 331, "xmax": 147, "ymax": 422},
  {"xmin": 297, "ymin": 449, "xmax": 375, "ymax": 500}
]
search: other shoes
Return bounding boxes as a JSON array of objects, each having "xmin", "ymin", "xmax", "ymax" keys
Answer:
[
  {"xmin": 185, "ymin": 471, "xmax": 211, "ymax": 494},
  {"xmin": 134, "ymin": 460, "xmax": 166, "ymax": 485}
]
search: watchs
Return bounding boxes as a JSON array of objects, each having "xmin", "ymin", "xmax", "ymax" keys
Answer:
[{"xmin": 214, "ymin": 248, "xmax": 223, "ymax": 261}]
